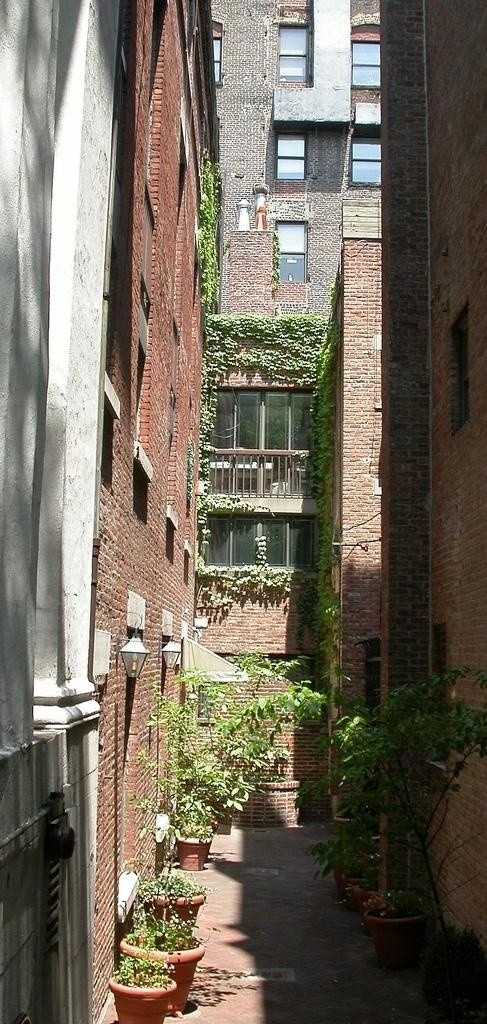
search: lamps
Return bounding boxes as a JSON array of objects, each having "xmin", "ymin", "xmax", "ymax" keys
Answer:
[
  {"xmin": 114, "ymin": 612, "xmax": 150, "ymax": 679},
  {"xmin": 158, "ymin": 624, "xmax": 181, "ymax": 669}
]
